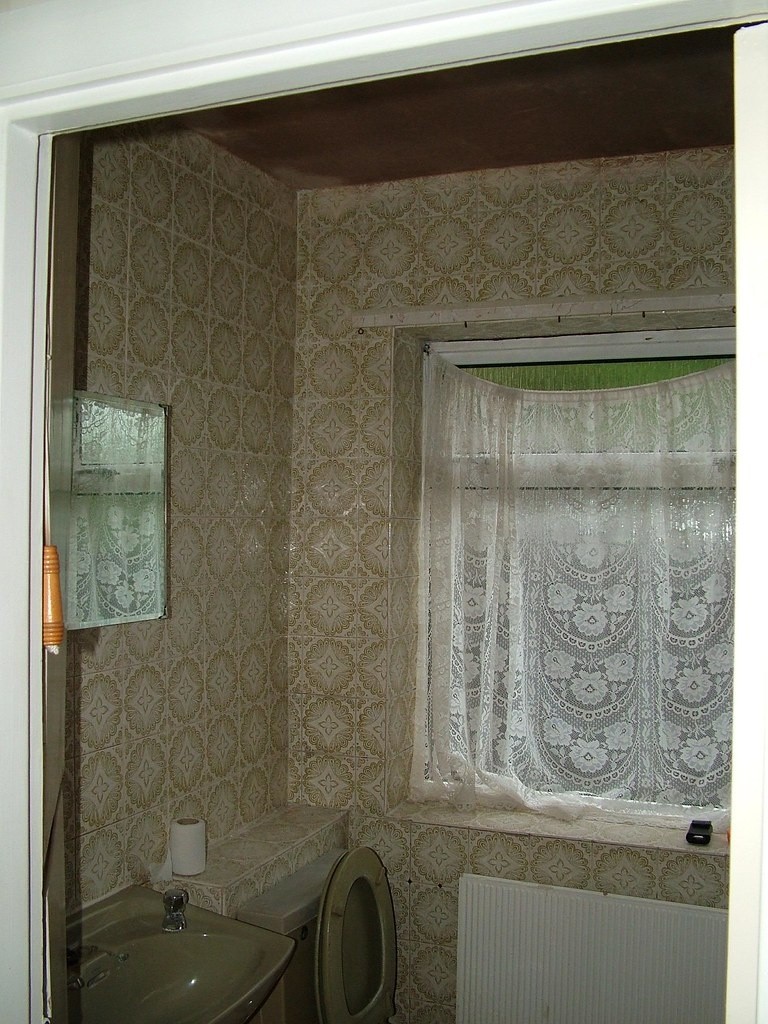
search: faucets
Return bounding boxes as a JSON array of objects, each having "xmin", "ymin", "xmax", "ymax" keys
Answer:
[{"xmin": 83, "ymin": 944, "xmax": 129, "ymax": 963}]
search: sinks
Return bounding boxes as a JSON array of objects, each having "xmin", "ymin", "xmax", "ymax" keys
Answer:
[{"xmin": 69, "ymin": 883, "xmax": 299, "ymax": 1024}]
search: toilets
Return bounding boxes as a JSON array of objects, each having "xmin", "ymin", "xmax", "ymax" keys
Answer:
[{"xmin": 236, "ymin": 845, "xmax": 397, "ymax": 1024}]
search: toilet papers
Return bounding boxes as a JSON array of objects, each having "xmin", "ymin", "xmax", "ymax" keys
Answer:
[{"xmin": 150, "ymin": 816, "xmax": 207, "ymax": 883}]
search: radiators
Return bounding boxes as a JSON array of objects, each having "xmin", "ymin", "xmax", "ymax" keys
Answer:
[{"xmin": 453, "ymin": 873, "xmax": 729, "ymax": 1024}]
